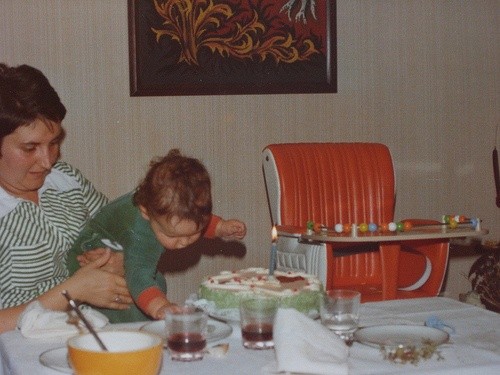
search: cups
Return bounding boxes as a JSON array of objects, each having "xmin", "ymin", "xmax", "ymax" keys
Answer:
[
  {"xmin": 240, "ymin": 293, "xmax": 280, "ymax": 348},
  {"xmin": 164, "ymin": 306, "xmax": 208, "ymax": 361},
  {"xmin": 320, "ymin": 289, "xmax": 361, "ymax": 344}
]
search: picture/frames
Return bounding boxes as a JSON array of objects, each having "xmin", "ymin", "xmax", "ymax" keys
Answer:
[{"xmin": 128, "ymin": 0, "xmax": 337, "ymax": 97}]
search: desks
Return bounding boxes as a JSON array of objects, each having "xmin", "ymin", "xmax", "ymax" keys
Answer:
[{"xmin": 0, "ymin": 297, "xmax": 500, "ymax": 375}]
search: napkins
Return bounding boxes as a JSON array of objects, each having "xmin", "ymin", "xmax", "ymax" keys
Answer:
[{"xmin": 271, "ymin": 307, "xmax": 350, "ymax": 375}]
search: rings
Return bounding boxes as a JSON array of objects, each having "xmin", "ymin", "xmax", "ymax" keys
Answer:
[{"xmin": 114, "ymin": 293, "xmax": 121, "ymax": 303}]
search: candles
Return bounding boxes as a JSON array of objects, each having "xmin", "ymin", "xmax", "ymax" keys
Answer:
[{"xmin": 269, "ymin": 226, "xmax": 277, "ymax": 276}]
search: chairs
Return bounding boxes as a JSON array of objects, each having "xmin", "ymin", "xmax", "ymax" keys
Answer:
[{"xmin": 261, "ymin": 142, "xmax": 451, "ymax": 299}]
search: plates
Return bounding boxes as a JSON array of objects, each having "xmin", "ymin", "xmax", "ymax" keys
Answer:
[
  {"xmin": 38, "ymin": 345, "xmax": 72, "ymax": 374},
  {"xmin": 140, "ymin": 317, "xmax": 233, "ymax": 348},
  {"xmin": 354, "ymin": 324, "xmax": 450, "ymax": 349}
]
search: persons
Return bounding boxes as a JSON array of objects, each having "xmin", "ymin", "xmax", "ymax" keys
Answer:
[
  {"xmin": 65, "ymin": 149, "xmax": 247, "ymax": 328},
  {"xmin": 0, "ymin": 61, "xmax": 134, "ymax": 334}
]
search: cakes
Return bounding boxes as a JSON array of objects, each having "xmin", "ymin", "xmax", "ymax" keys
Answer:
[{"xmin": 197, "ymin": 267, "xmax": 324, "ymax": 318}]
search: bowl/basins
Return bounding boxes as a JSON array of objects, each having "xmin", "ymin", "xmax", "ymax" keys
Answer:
[{"xmin": 66, "ymin": 329, "xmax": 163, "ymax": 375}]
